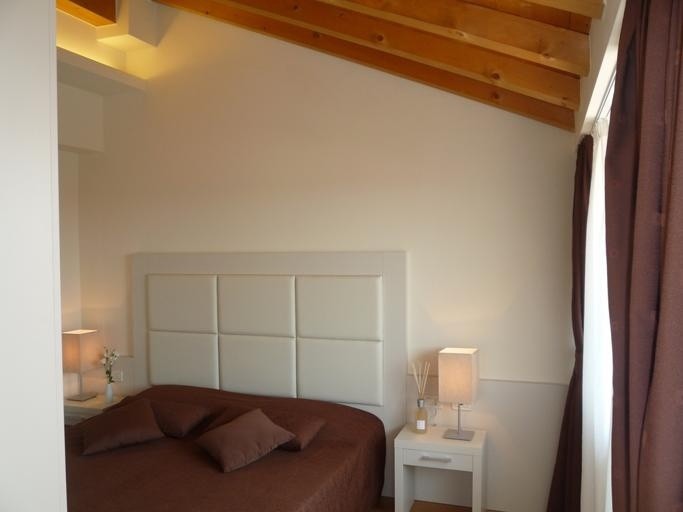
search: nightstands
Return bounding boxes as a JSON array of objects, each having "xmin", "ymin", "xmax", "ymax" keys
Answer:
[
  {"xmin": 63, "ymin": 394, "xmax": 124, "ymax": 426},
  {"xmin": 393, "ymin": 421, "xmax": 487, "ymax": 512}
]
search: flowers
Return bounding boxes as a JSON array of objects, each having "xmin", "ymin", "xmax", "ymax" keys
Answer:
[{"xmin": 98, "ymin": 345, "xmax": 119, "ymax": 383}]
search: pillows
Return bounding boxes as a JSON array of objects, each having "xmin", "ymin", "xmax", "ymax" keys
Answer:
[{"xmin": 69, "ymin": 394, "xmax": 325, "ymax": 474}]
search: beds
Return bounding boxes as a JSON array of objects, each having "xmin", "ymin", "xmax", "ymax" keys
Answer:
[{"xmin": 63, "ymin": 246, "xmax": 411, "ymax": 512}]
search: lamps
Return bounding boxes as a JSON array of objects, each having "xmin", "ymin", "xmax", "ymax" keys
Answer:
[
  {"xmin": 62, "ymin": 328, "xmax": 102, "ymax": 401},
  {"xmin": 437, "ymin": 346, "xmax": 478, "ymax": 442}
]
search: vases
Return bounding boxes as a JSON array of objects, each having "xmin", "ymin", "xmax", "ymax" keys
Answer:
[{"xmin": 104, "ymin": 383, "xmax": 113, "ymax": 404}]
